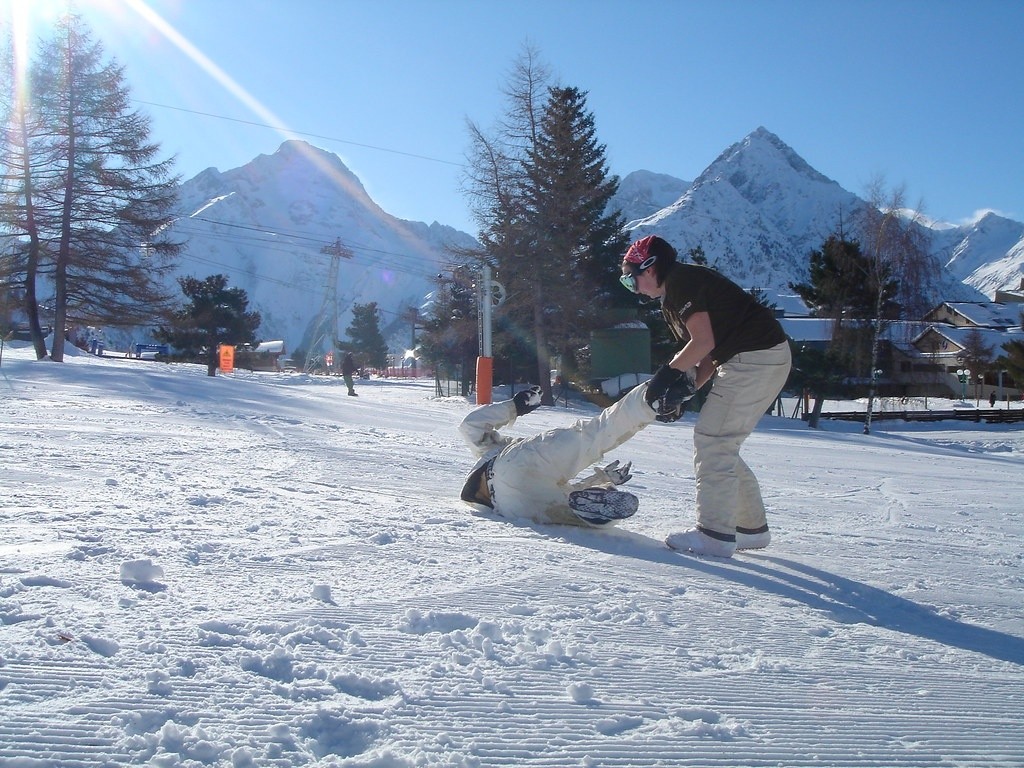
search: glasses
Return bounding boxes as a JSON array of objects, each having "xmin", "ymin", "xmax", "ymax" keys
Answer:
[{"xmin": 620, "ymin": 273, "xmax": 637, "ymax": 292}]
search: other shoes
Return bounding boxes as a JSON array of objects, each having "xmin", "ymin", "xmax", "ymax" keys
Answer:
[
  {"xmin": 665, "ymin": 530, "xmax": 736, "ymax": 558},
  {"xmin": 569, "ymin": 490, "xmax": 639, "ymax": 521},
  {"xmin": 348, "ymin": 394, "xmax": 358, "ymax": 396},
  {"xmin": 657, "ymin": 371, "xmax": 697, "ymax": 415},
  {"xmin": 736, "ymin": 530, "xmax": 771, "ymax": 550}
]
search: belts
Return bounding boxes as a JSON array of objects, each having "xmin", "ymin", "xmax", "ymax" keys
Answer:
[{"xmin": 486, "ymin": 456, "xmax": 497, "ymax": 509}]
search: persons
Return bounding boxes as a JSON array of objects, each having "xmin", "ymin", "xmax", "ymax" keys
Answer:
[
  {"xmin": 342, "ymin": 352, "xmax": 359, "ymax": 396},
  {"xmin": 989, "ymin": 390, "xmax": 997, "ymax": 407},
  {"xmin": 619, "ymin": 236, "xmax": 792, "ymax": 558},
  {"xmin": 459, "ymin": 351, "xmax": 698, "ymax": 528}
]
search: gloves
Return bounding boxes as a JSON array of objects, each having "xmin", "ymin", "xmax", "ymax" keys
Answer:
[
  {"xmin": 656, "ymin": 401, "xmax": 688, "ymax": 423},
  {"xmin": 513, "ymin": 386, "xmax": 543, "ymax": 416},
  {"xmin": 603, "ymin": 460, "xmax": 632, "ymax": 486},
  {"xmin": 645, "ymin": 362, "xmax": 682, "ymax": 412}
]
